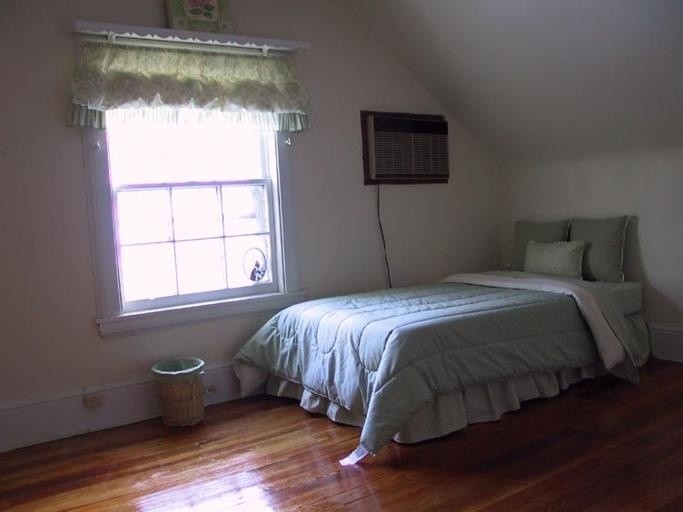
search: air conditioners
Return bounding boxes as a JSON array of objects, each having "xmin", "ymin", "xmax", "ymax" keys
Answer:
[{"xmin": 367, "ymin": 112, "xmax": 449, "ymax": 182}]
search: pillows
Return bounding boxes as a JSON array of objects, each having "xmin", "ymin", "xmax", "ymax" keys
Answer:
[{"xmin": 500, "ymin": 215, "xmax": 630, "ymax": 281}]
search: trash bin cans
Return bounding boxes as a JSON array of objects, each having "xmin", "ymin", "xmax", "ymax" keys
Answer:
[{"xmin": 149, "ymin": 357, "xmax": 207, "ymax": 428}]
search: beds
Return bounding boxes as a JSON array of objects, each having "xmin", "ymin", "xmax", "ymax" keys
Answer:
[{"xmin": 270, "ymin": 272, "xmax": 644, "ymax": 445}]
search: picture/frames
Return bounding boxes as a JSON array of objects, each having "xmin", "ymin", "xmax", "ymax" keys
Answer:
[{"xmin": 165, "ymin": 0, "xmax": 235, "ymax": 34}]
